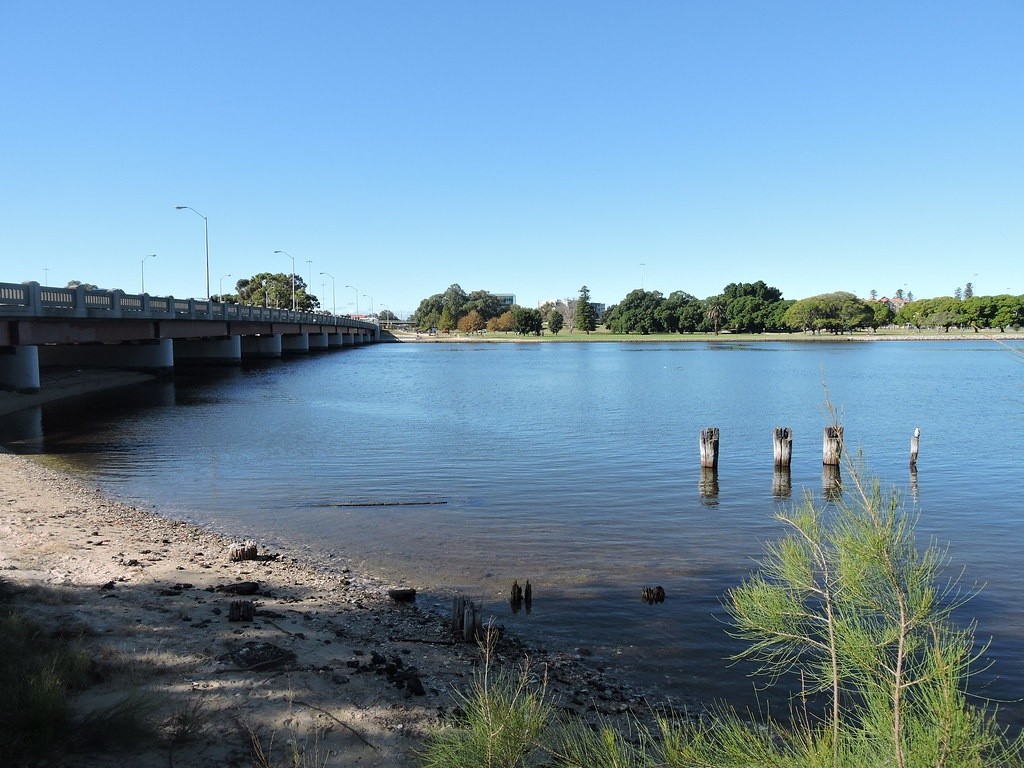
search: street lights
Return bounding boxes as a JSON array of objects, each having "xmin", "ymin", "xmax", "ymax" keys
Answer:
[
  {"xmin": 141, "ymin": 254, "xmax": 157, "ymax": 292},
  {"xmin": 306, "ymin": 259, "xmax": 312, "ymax": 295},
  {"xmin": 363, "ymin": 295, "xmax": 374, "ymax": 313},
  {"xmin": 174, "ymin": 205, "xmax": 209, "ymax": 300},
  {"xmin": 345, "ymin": 285, "xmax": 358, "ymax": 314},
  {"xmin": 320, "ymin": 273, "xmax": 335, "ymax": 315},
  {"xmin": 274, "ymin": 250, "xmax": 295, "ymax": 311},
  {"xmin": 380, "ymin": 304, "xmax": 390, "ymax": 326},
  {"xmin": 219, "ymin": 274, "xmax": 231, "ymax": 302}
]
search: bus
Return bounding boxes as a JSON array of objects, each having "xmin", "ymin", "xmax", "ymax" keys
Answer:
[{"xmin": 359, "ymin": 317, "xmax": 378, "ymax": 324}]
